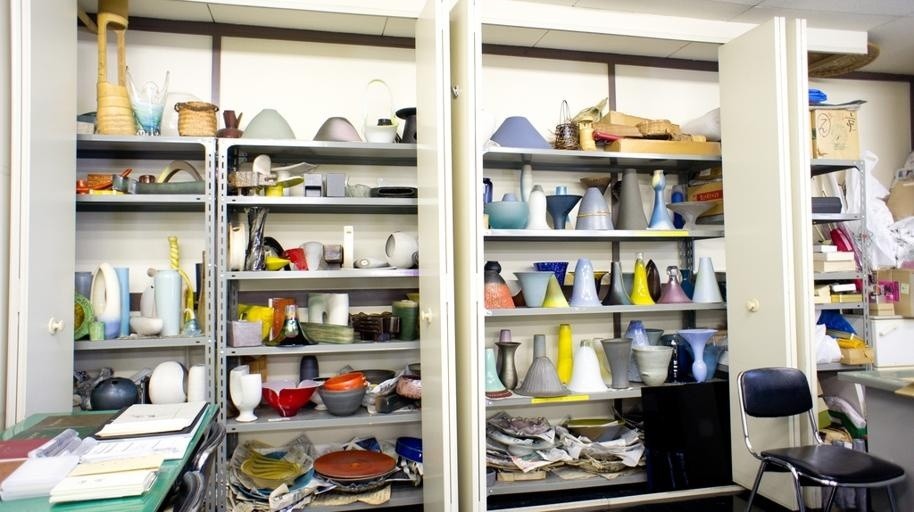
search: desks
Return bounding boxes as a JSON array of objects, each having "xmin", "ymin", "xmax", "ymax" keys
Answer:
[
  {"xmin": 831, "ymin": 369, "xmax": 914, "ymax": 512},
  {"xmin": 0, "ymin": 402, "xmax": 221, "ymax": 512}
]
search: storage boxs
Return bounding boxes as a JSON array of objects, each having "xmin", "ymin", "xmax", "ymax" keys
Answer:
[
  {"xmin": 886, "ymin": 176, "xmax": 914, "ymax": 222},
  {"xmin": 808, "ymin": 109, "xmax": 861, "ymax": 161},
  {"xmin": 877, "ymin": 269, "xmax": 914, "ymax": 317}
]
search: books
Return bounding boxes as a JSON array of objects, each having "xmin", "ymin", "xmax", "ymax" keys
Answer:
[
  {"xmin": 814, "ymin": 243, "xmax": 896, "ymax": 318},
  {"xmin": 0, "ymin": 453, "xmax": 164, "ymax": 505}
]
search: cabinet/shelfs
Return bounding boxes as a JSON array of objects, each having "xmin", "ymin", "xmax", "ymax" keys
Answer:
[
  {"xmin": 7, "ymin": 0, "xmax": 459, "ymax": 512},
  {"xmin": 449, "ymin": 0, "xmax": 802, "ymax": 512},
  {"xmin": 784, "ymin": 18, "xmax": 871, "ymax": 510}
]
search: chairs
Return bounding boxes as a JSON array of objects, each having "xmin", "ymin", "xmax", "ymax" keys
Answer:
[
  {"xmin": 738, "ymin": 367, "xmax": 906, "ymax": 512},
  {"xmin": 185, "ymin": 423, "xmax": 226, "ymax": 472},
  {"xmin": 153, "ymin": 471, "xmax": 206, "ymax": 512}
]
